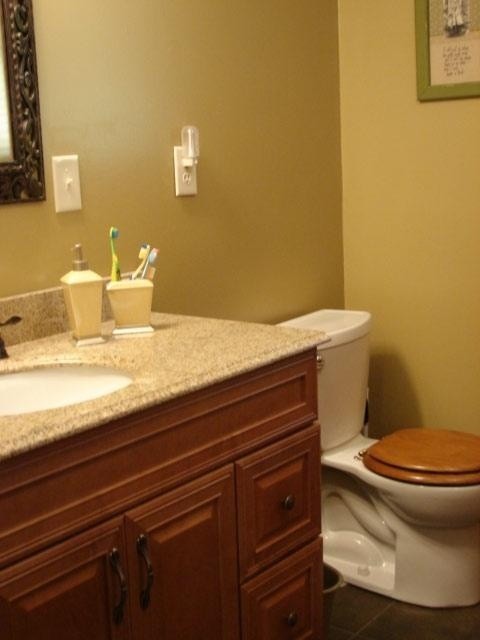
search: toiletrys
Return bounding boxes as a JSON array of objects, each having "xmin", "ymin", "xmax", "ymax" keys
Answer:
[{"xmin": 60, "ymin": 245, "xmax": 107, "ymax": 346}]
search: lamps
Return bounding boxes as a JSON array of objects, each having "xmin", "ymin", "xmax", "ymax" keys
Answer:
[{"xmin": 180, "ymin": 125, "xmax": 200, "ymax": 169}]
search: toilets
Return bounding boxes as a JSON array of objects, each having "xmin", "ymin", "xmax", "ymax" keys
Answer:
[{"xmin": 276, "ymin": 308, "xmax": 479, "ymax": 609}]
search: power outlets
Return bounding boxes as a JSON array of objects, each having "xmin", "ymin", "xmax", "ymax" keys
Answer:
[{"xmin": 174, "ymin": 146, "xmax": 198, "ymax": 196}]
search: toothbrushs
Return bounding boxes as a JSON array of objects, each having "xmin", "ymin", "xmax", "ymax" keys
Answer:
[
  {"xmin": 131, "ymin": 244, "xmax": 158, "ymax": 281},
  {"xmin": 109, "ymin": 226, "xmax": 121, "ymax": 282}
]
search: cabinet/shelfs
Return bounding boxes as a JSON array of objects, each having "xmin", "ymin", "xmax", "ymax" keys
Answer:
[{"xmin": 0, "ymin": 346, "xmax": 324, "ymax": 640}]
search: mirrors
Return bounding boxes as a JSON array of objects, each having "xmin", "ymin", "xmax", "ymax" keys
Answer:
[{"xmin": 0, "ymin": 0, "xmax": 47, "ymax": 204}]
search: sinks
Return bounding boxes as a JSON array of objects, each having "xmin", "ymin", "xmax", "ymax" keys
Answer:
[{"xmin": 0, "ymin": 361, "xmax": 137, "ymax": 417}]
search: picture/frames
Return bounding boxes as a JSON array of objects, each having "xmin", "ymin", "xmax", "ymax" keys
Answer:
[{"xmin": 414, "ymin": 0, "xmax": 480, "ymax": 102}]
